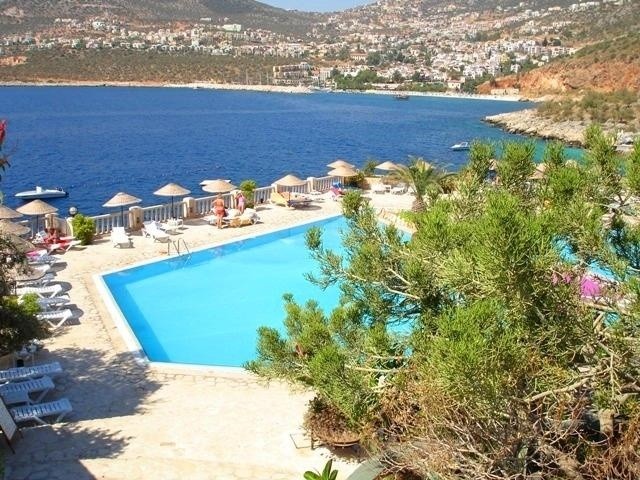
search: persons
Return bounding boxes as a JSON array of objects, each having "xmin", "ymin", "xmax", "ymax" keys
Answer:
[
  {"xmin": 211, "ymin": 194, "xmax": 225, "ymax": 229},
  {"xmin": 235, "ymin": 190, "xmax": 247, "ymax": 213}
]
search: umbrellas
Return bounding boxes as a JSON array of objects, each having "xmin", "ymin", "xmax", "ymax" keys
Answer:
[
  {"xmin": 15, "ymin": 199, "xmax": 60, "ymax": 232},
  {"xmin": 375, "ymin": 161, "xmax": 397, "ymax": 173},
  {"xmin": 275, "ymin": 174, "xmax": 307, "ymax": 201},
  {"xmin": 102, "ymin": 192, "xmax": 142, "ymax": 227},
  {"xmin": 326, "ymin": 159, "xmax": 355, "ymax": 169},
  {"xmin": 152, "ymin": 183, "xmax": 192, "ymax": 217},
  {"xmin": 0, "ymin": 205, "xmax": 45, "ymax": 295},
  {"xmin": 202, "ymin": 179, "xmax": 237, "ymax": 193},
  {"xmin": 328, "ymin": 167, "xmax": 359, "ymax": 185}
]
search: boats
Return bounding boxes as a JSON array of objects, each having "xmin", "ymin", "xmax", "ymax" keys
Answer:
[
  {"xmin": 451, "ymin": 140, "xmax": 470, "ymax": 151},
  {"xmin": 12, "ymin": 184, "xmax": 72, "ymax": 201},
  {"xmin": 395, "ymin": 94, "xmax": 411, "ymax": 100}
]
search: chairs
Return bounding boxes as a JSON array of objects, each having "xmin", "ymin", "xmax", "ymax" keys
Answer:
[
  {"xmin": 209, "ymin": 184, "xmax": 408, "ymax": 230},
  {"xmin": 1, "ymin": 230, "xmax": 83, "ymax": 428},
  {"xmin": 111, "ymin": 219, "xmax": 182, "ymax": 248}
]
